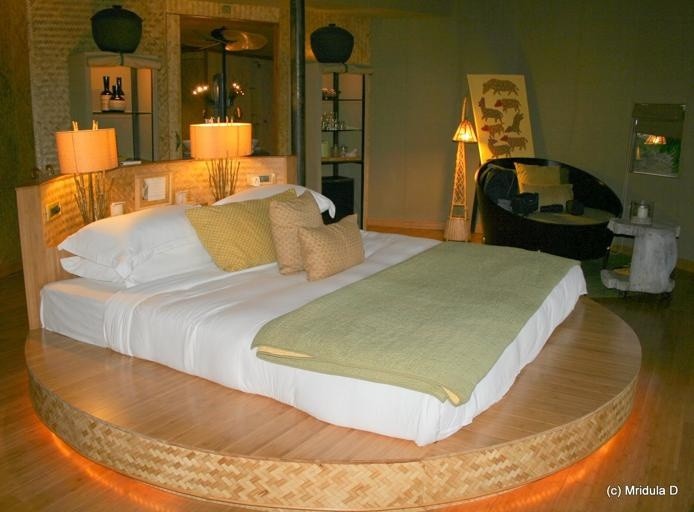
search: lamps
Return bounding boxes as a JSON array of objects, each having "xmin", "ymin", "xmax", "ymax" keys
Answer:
[
  {"xmin": 191, "ymin": 84, "xmax": 215, "ymax": 108},
  {"xmin": 189, "ymin": 123, "xmax": 253, "ymax": 201},
  {"xmin": 227, "ymin": 81, "xmax": 247, "ymax": 108},
  {"xmin": 54, "ymin": 128, "xmax": 119, "ymax": 222},
  {"xmin": 443, "ymin": 97, "xmax": 478, "ymax": 243}
]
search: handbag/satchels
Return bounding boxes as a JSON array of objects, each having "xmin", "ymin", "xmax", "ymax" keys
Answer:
[{"xmin": 513, "ymin": 191, "xmax": 541, "ymax": 219}]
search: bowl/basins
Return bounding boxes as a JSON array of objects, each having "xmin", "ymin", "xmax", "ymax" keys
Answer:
[{"xmin": 321, "ymin": 90, "xmax": 341, "ymax": 98}]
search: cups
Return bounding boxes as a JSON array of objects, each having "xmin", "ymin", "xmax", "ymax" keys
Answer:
[{"xmin": 321, "ymin": 112, "xmax": 348, "ymax": 158}]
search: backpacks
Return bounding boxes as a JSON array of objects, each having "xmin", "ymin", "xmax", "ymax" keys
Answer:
[{"xmin": 477, "ymin": 162, "xmax": 518, "ymax": 204}]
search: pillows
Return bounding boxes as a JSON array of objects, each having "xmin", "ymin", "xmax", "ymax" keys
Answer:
[
  {"xmin": 58, "ymin": 240, "xmax": 213, "ymax": 289},
  {"xmin": 56, "ymin": 204, "xmax": 197, "ymax": 280},
  {"xmin": 487, "ymin": 163, "xmax": 518, "ymax": 199},
  {"xmin": 210, "ymin": 183, "xmax": 337, "ymax": 221},
  {"xmin": 185, "ymin": 190, "xmax": 296, "ymax": 274},
  {"xmin": 560, "ymin": 168, "xmax": 569, "ymax": 185},
  {"xmin": 521, "ymin": 183, "xmax": 575, "ymax": 212},
  {"xmin": 297, "ymin": 213, "xmax": 363, "ymax": 282},
  {"xmin": 269, "ymin": 190, "xmax": 326, "ymax": 276},
  {"xmin": 514, "ymin": 161, "xmax": 561, "ymax": 197}
]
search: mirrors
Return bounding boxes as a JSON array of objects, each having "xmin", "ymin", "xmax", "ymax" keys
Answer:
[{"xmin": 181, "ymin": 46, "xmax": 274, "ymax": 160}]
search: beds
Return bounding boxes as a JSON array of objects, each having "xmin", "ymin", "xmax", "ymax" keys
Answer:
[
  {"xmin": 13, "ymin": 156, "xmax": 643, "ymax": 512},
  {"xmin": 607, "ymin": 217, "xmax": 681, "ymax": 239}
]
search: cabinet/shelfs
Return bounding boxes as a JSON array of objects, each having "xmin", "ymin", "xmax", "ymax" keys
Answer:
[
  {"xmin": 321, "ymin": 63, "xmax": 365, "ymax": 230},
  {"xmin": 67, "ymin": 51, "xmax": 162, "ymax": 162}
]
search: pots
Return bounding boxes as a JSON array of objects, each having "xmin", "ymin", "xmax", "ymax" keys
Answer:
[
  {"xmin": 90, "ymin": 5, "xmax": 144, "ymax": 53},
  {"xmin": 310, "ymin": 24, "xmax": 354, "ymax": 63}
]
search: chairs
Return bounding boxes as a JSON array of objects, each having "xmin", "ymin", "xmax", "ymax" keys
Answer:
[
  {"xmin": 469, "ymin": 157, "xmax": 623, "ymax": 271},
  {"xmin": 600, "ymin": 230, "xmax": 678, "ymax": 309}
]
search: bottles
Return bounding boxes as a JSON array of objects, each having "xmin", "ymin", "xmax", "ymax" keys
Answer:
[
  {"xmin": 637, "ymin": 206, "xmax": 648, "ymax": 218},
  {"xmin": 101, "ymin": 74, "xmax": 126, "ymax": 113}
]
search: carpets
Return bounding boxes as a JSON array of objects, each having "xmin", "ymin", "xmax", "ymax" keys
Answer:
[{"xmin": 578, "ymin": 254, "xmax": 641, "ymax": 299}]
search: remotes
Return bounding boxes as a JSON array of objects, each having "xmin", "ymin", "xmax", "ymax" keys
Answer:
[{"xmin": 541, "ymin": 205, "xmax": 563, "ymax": 212}]
